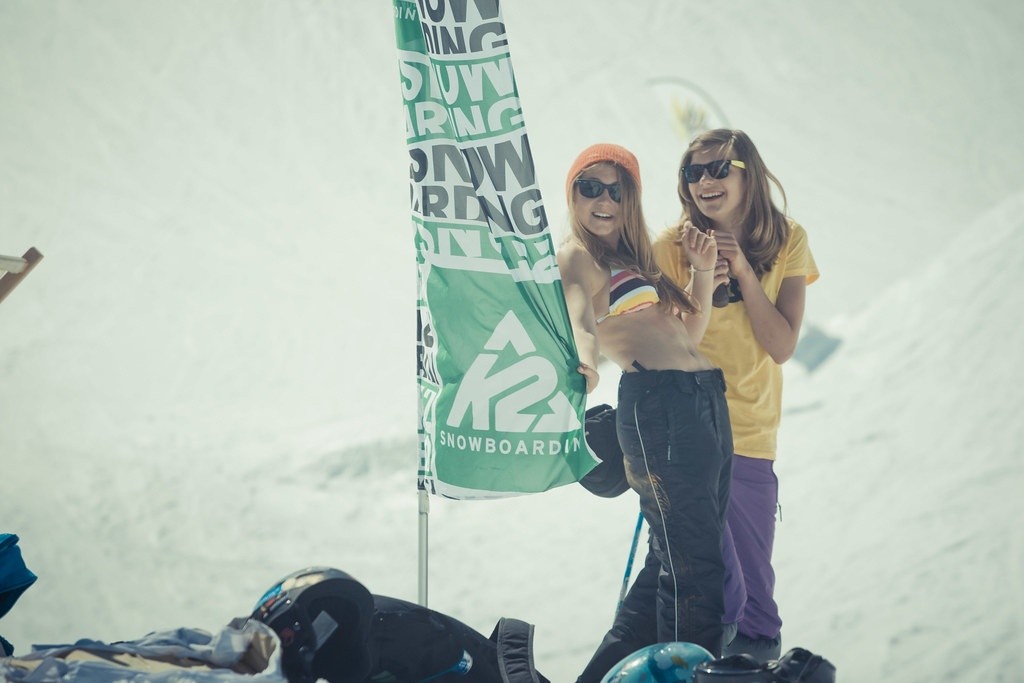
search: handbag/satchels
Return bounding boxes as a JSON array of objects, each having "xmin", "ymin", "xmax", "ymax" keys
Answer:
[{"xmin": 562, "ymin": 404, "xmax": 632, "ymax": 498}]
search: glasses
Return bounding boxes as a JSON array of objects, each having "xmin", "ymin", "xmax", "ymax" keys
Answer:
[
  {"xmin": 681, "ymin": 159, "xmax": 746, "ymax": 183},
  {"xmin": 574, "ymin": 178, "xmax": 636, "ymax": 204}
]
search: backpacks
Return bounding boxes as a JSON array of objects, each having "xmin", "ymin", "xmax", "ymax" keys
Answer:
[{"xmin": 372, "ymin": 594, "xmax": 551, "ymax": 683}]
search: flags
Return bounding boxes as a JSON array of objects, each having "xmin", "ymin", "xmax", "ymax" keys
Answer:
[{"xmin": 394, "ymin": 1, "xmax": 603, "ymax": 500}]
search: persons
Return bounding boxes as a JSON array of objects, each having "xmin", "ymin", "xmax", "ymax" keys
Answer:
[
  {"xmin": 557, "ymin": 143, "xmax": 734, "ymax": 683},
  {"xmin": 648, "ymin": 128, "xmax": 819, "ymax": 663}
]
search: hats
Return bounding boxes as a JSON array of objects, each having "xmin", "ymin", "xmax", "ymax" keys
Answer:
[{"xmin": 565, "ymin": 143, "xmax": 642, "ymax": 205}]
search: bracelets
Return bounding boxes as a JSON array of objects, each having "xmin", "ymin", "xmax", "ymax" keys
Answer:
[{"xmin": 692, "ymin": 267, "xmax": 713, "ymax": 272}]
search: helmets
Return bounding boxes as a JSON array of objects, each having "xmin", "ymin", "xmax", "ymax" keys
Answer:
[
  {"xmin": 690, "ymin": 648, "xmax": 836, "ymax": 683},
  {"xmin": 252, "ymin": 566, "xmax": 375, "ymax": 683},
  {"xmin": 599, "ymin": 641, "xmax": 716, "ymax": 683}
]
